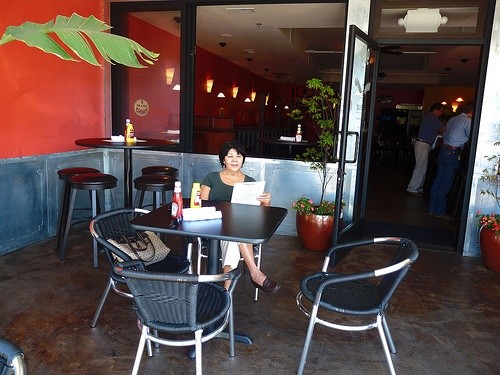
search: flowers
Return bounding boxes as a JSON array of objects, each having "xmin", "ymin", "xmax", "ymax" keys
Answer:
[
  {"xmin": 475, "ymin": 208, "xmax": 500, "ymax": 234},
  {"xmin": 288, "ymin": 193, "xmax": 346, "ymax": 218}
]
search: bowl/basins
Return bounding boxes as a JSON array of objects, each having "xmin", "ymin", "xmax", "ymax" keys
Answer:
[{"xmin": 111, "ymin": 135, "xmax": 124, "ymax": 142}]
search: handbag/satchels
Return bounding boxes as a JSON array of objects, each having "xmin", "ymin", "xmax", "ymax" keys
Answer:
[{"xmin": 107, "ymin": 231, "xmax": 171, "ymax": 266}]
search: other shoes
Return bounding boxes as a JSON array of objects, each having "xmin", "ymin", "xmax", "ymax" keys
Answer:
[
  {"xmin": 428, "ymin": 211, "xmax": 452, "ymax": 220},
  {"xmin": 406, "ymin": 190, "xmax": 425, "ymax": 197}
]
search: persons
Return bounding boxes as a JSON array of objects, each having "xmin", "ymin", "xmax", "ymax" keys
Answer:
[
  {"xmin": 199, "ymin": 141, "xmax": 281, "ymax": 294},
  {"xmin": 429, "ymin": 101, "xmax": 476, "ymax": 220},
  {"xmin": 407, "ymin": 102, "xmax": 446, "ymax": 197}
]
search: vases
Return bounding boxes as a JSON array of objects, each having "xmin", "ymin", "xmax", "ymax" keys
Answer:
[
  {"xmin": 295, "ymin": 206, "xmax": 344, "ymax": 252},
  {"xmin": 479, "ymin": 226, "xmax": 500, "ymax": 273}
]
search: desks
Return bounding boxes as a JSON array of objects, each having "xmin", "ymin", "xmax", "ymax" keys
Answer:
[
  {"xmin": 74, "ymin": 137, "xmax": 180, "ymax": 208},
  {"xmin": 256, "ymin": 139, "xmax": 310, "ymax": 160},
  {"xmin": 128, "ymin": 198, "xmax": 288, "ymax": 359}
]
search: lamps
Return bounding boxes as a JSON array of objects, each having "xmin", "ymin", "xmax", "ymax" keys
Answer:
[
  {"xmin": 166, "ymin": 68, "xmax": 175, "ymax": 85},
  {"xmin": 206, "ymin": 80, "xmax": 269, "ymax": 105}
]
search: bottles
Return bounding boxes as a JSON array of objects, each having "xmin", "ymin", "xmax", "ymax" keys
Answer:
[
  {"xmin": 189, "ymin": 182, "xmax": 202, "ymax": 208},
  {"xmin": 296, "ymin": 128, "xmax": 302, "ymax": 142},
  {"xmin": 124, "ymin": 119, "xmax": 134, "ymax": 145},
  {"xmin": 172, "ymin": 181, "xmax": 183, "ymax": 221}
]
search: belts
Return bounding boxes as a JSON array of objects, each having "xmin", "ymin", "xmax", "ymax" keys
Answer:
[
  {"xmin": 441, "ymin": 144, "xmax": 463, "ymax": 151},
  {"xmin": 416, "ymin": 138, "xmax": 432, "ymax": 147}
]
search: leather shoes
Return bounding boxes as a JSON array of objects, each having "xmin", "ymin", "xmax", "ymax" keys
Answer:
[{"xmin": 251, "ymin": 276, "xmax": 281, "ymax": 294}]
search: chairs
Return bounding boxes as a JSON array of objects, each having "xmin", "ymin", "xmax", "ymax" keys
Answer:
[
  {"xmin": 296, "ymin": 237, "xmax": 419, "ymax": 375},
  {"xmin": 90, "ymin": 204, "xmax": 262, "ymax": 375}
]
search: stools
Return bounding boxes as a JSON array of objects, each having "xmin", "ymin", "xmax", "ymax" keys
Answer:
[{"xmin": 55, "ymin": 166, "xmax": 180, "ymax": 268}]
